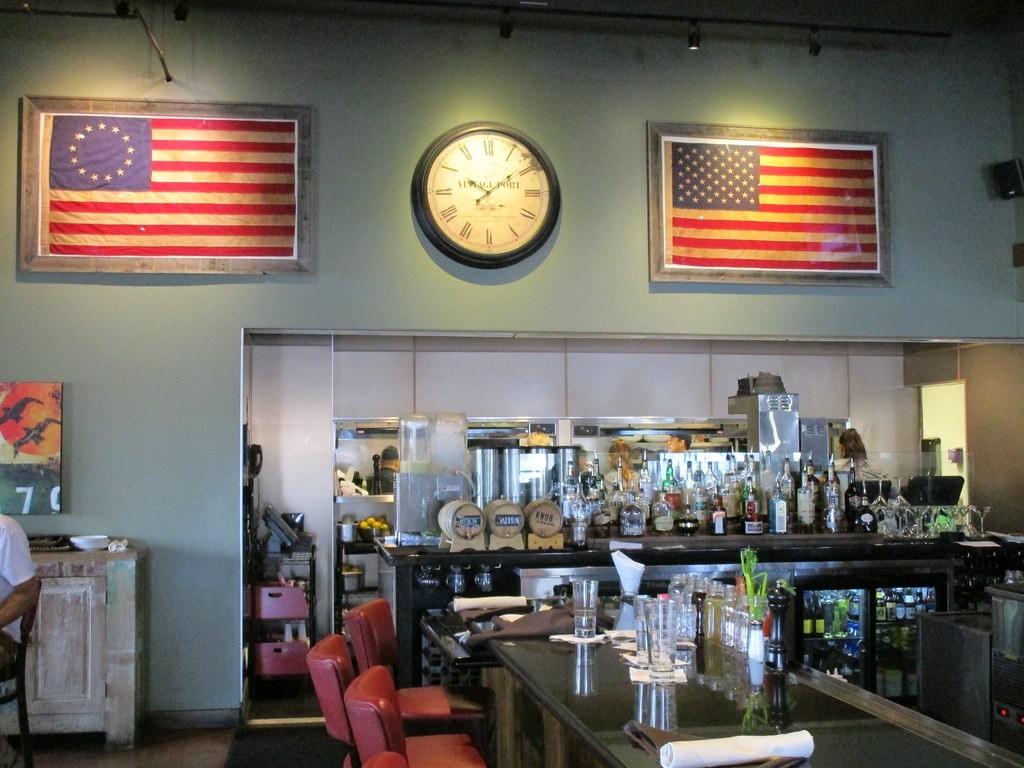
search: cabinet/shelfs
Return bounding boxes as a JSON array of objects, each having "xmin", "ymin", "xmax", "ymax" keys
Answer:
[
  {"xmin": 790, "ymin": 571, "xmax": 948, "ymax": 706},
  {"xmin": 249, "ymin": 544, "xmax": 316, "ymax": 696},
  {"xmin": 0, "ymin": 577, "xmax": 107, "ymax": 715},
  {"xmin": 336, "ymin": 541, "xmax": 377, "ymax": 646}
]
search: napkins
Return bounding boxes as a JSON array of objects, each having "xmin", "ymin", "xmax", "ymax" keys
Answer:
[
  {"xmin": 659, "ymin": 729, "xmax": 814, "ymax": 768},
  {"xmin": 612, "ymin": 549, "xmax": 645, "ymax": 596},
  {"xmin": 454, "ymin": 595, "xmax": 527, "ymax": 612},
  {"xmin": 549, "ymin": 634, "xmax": 691, "ymax": 683}
]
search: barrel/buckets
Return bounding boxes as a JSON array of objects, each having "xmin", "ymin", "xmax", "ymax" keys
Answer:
[{"xmin": 438, "ymin": 495, "xmax": 563, "ymax": 539}]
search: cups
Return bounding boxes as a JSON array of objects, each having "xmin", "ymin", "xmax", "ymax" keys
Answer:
[
  {"xmin": 865, "ymin": 480, "xmax": 892, "ymax": 504},
  {"xmin": 572, "ymin": 580, "xmax": 598, "ymax": 638},
  {"xmin": 678, "ymin": 604, "xmax": 697, "ymax": 640}
]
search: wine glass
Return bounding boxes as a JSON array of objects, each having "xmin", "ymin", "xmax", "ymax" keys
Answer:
[{"xmin": 863, "ymin": 471, "xmax": 990, "ymax": 538}]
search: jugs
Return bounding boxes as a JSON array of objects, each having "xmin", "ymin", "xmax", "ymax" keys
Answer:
[{"xmin": 417, "ymin": 565, "xmax": 491, "ymax": 593}]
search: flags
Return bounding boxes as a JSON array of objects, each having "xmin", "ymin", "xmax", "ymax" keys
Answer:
[
  {"xmin": 41, "ymin": 114, "xmax": 296, "ymax": 256},
  {"xmin": 665, "ymin": 141, "xmax": 878, "ymax": 270}
]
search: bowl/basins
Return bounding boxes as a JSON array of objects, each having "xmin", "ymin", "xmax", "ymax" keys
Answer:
[
  {"xmin": 336, "ymin": 520, "xmax": 359, "ymax": 543},
  {"xmin": 69, "ymin": 535, "xmax": 110, "ymax": 551},
  {"xmin": 343, "ymin": 571, "xmax": 361, "ymax": 591},
  {"xmin": 357, "ymin": 528, "xmax": 390, "ymax": 543}
]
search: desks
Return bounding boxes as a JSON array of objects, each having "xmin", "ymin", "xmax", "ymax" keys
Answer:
[{"xmin": 446, "ymin": 599, "xmax": 1024, "ymax": 768}]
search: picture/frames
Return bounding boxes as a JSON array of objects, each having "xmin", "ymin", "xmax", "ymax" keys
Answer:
[
  {"xmin": 646, "ymin": 121, "xmax": 895, "ymax": 289},
  {"xmin": 18, "ymin": 96, "xmax": 318, "ymax": 276}
]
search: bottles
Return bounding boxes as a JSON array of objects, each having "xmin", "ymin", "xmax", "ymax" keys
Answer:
[
  {"xmin": 668, "ymin": 573, "xmax": 709, "ymax": 635},
  {"xmin": 803, "ymin": 590, "xmax": 860, "ymax": 638},
  {"xmin": 633, "ymin": 594, "xmax": 677, "ymax": 681},
  {"xmin": 748, "ymin": 619, "xmax": 764, "ymax": 662},
  {"xmin": 875, "ymin": 587, "xmax": 936, "ymax": 620},
  {"xmin": 361, "ymin": 478, "xmax": 367, "ymax": 490},
  {"xmin": 673, "ymin": 465, "xmax": 685, "ymax": 482},
  {"xmin": 1005, "ymin": 570, "xmax": 1024, "ymax": 584},
  {"xmin": 694, "ymin": 459, "xmax": 704, "ymax": 478},
  {"xmin": 563, "ymin": 445, "xmax": 763, "ymax": 550},
  {"xmin": 712, "ymin": 461, "xmax": 722, "ymax": 487},
  {"xmin": 770, "ymin": 449, "xmax": 877, "ymax": 538},
  {"xmin": 953, "ymin": 574, "xmax": 999, "ymax": 605},
  {"xmin": 704, "ymin": 580, "xmax": 759, "ymax": 652},
  {"xmin": 876, "ymin": 625, "xmax": 917, "ymax": 657},
  {"xmin": 803, "ymin": 622, "xmax": 861, "ymax": 684}
]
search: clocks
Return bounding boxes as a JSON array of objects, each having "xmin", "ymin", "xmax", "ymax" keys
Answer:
[{"xmin": 412, "ymin": 122, "xmax": 560, "ymax": 268}]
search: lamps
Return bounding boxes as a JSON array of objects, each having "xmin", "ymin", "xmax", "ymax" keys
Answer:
[
  {"xmin": 809, "ymin": 26, "xmax": 821, "ymax": 55},
  {"xmin": 687, "ymin": 20, "xmax": 700, "ymax": 50},
  {"xmin": 497, "ymin": 8, "xmax": 514, "ymax": 38},
  {"xmin": 174, "ymin": 0, "xmax": 189, "ymax": 23}
]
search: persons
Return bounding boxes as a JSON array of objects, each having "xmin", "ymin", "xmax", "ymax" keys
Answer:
[
  {"xmin": 834, "ymin": 428, "xmax": 898, "ymax": 497},
  {"xmin": 365, "ymin": 446, "xmax": 399, "ymax": 495},
  {"xmin": 604, "ymin": 439, "xmax": 640, "ymax": 494},
  {"xmin": 667, "ymin": 434, "xmax": 692, "ymax": 452},
  {"xmin": 0, "ymin": 513, "xmax": 39, "ymax": 682},
  {"xmin": 527, "ymin": 431, "xmax": 550, "ymax": 446}
]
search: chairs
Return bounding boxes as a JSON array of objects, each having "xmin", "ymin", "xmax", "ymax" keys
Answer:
[
  {"xmin": 305, "ymin": 597, "xmax": 488, "ymax": 768},
  {"xmin": 0, "ymin": 577, "xmax": 42, "ymax": 768}
]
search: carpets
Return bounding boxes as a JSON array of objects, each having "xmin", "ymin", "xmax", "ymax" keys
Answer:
[{"xmin": 224, "ymin": 726, "xmax": 348, "ymax": 768}]
